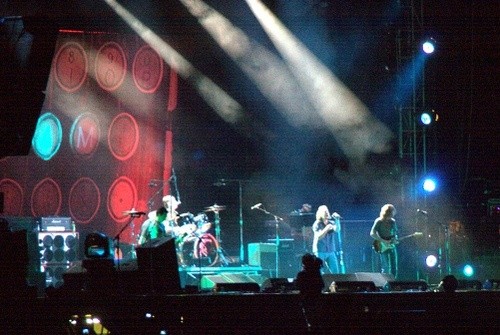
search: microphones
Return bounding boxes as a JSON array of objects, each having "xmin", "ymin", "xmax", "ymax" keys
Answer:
[
  {"xmin": 417, "ymin": 209, "xmax": 426, "ymax": 214},
  {"xmin": 251, "ymin": 203, "xmax": 262, "ymax": 210},
  {"xmin": 131, "ymin": 212, "xmax": 146, "ymax": 215},
  {"xmin": 173, "ymin": 178, "xmax": 180, "ymax": 201}
]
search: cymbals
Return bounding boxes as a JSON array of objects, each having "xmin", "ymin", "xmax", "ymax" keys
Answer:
[
  {"xmin": 120, "ymin": 210, "xmax": 146, "ymax": 217},
  {"xmin": 202, "ymin": 205, "xmax": 226, "ymax": 212},
  {"xmin": 149, "ymin": 211, "xmax": 175, "ymax": 220}
]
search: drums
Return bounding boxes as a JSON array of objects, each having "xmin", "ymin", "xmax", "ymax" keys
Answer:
[
  {"xmin": 180, "ymin": 234, "xmax": 218, "ymax": 267},
  {"xmin": 174, "ymin": 212, "xmax": 197, "ymax": 234},
  {"xmin": 193, "ymin": 213, "xmax": 212, "ymax": 232}
]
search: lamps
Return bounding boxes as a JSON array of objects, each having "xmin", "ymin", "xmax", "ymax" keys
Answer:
[
  {"xmin": 420, "ymin": 108, "xmax": 435, "ymax": 124},
  {"xmin": 84, "ymin": 231, "xmax": 115, "ymax": 258},
  {"xmin": 422, "ymin": 36, "xmax": 437, "ymax": 54}
]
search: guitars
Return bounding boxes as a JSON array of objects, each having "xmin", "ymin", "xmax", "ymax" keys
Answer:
[{"xmin": 374, "ymin": 232, "xmax": 423, "ymax": 253}]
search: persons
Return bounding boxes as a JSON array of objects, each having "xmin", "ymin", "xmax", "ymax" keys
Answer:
[
  {"xmin": 140, "ymin": 195, "xmax": 180, "ymax": 242},
  {"xmin": 296, "ymin": 254, "xmax": 325, "ymax": 305},
  {"xmin": 370, "ymin": 204, "xmax": 400, "ymax": 280},
  {"xmin": 312, "ymin": 204, "xmax": 338, "ymax": 273}
]
